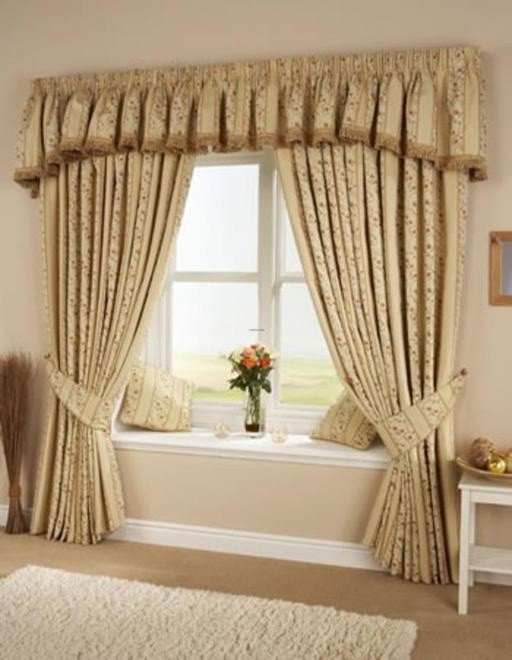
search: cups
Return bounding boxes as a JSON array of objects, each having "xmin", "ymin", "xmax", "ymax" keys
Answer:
[
  {"xmin": 272, "ymin": 426, "xmax": 285, "ymax": 442},
  {"xmin": 215, "ymin": 422, "xmax": 228, "ymax": 438}
]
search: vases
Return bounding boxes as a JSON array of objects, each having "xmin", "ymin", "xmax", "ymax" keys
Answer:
[{"xmin": 243, "ymin": 385, "xmax": 265, "ymax": 439}]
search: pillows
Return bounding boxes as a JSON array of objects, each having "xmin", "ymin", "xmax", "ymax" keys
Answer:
[
  {"xmin": 309, "ymin": 387, "xmax": 379, "ymax": 451},
  {"xmin": 117, "ymin": 365, "xmax": 195, "ymax": 433}
]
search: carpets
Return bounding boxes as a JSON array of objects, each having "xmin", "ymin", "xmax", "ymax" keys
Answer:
[{"xmin": 0, "ymin": 562, "xmax": 418, "ymax": 660}]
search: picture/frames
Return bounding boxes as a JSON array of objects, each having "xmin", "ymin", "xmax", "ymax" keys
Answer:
[{"xmin": 489, "ymin": 231, "xmax": 512, "ymax": 306}]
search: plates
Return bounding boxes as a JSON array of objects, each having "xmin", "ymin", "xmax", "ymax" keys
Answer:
[{"xmin": 454, "ymin": 454, "xmax": 512, "ymax": 481}]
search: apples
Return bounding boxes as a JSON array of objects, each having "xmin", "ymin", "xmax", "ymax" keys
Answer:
[{"xmin": 474, "ymin": 449, "xmax": 512, "ymax": 473}]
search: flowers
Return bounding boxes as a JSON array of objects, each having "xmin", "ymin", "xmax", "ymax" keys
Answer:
[{"xmin": 222, "ymin": 342, "xmax": 276, "ymax": 394}]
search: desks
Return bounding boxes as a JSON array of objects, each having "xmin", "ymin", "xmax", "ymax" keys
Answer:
[{"xmin": 456, "ymin": 472, "xmax": 512, "ymax": 614}]
